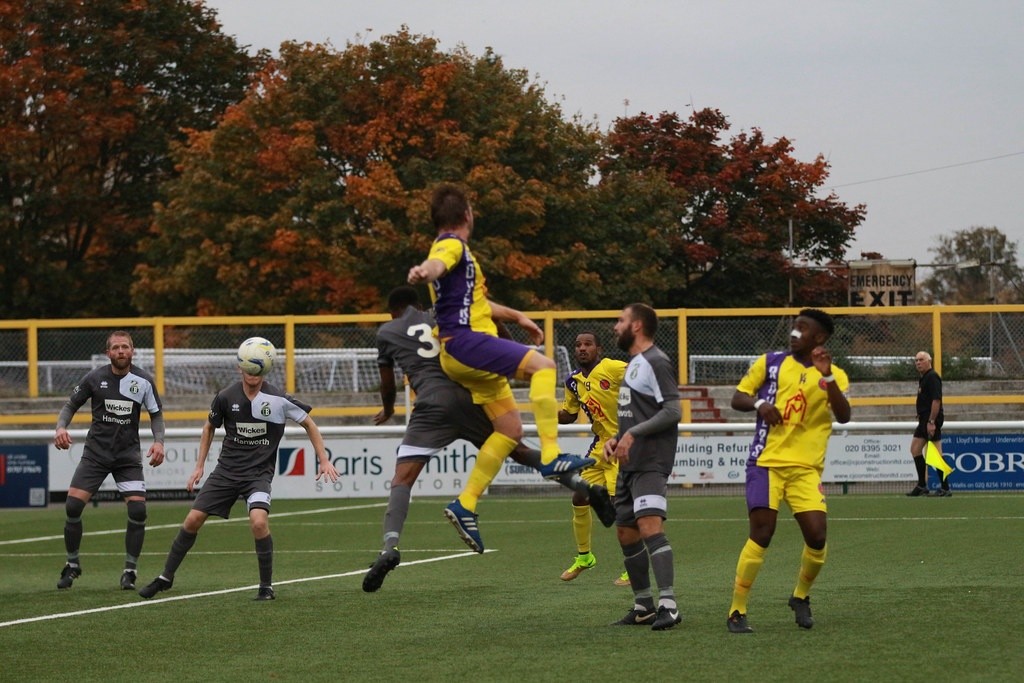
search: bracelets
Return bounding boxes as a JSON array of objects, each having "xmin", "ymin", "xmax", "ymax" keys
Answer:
[
  {"xmin": 754, "ymin": 399, "xmax": 766, "ymax": 410},
  {"xmin": 822, "ymin": 372, "xmax": 834, "ymax": 382}
]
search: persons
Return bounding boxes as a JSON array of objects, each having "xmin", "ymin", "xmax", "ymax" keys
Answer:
[
  {"xmin": 362, "ymin": 288, "xmax": 616, "ymax": 593},
  {"xmin": 906, "ymin": 351, "xmax": 952, "ymax": 497},
  {"xmin": 603, "ymin": 305, "xmax": 681, "ymax": 631},
  {"xmin": 409, "ymin": 186, "xmax": 596, "ymax": 556},
  {"xmin": 54, "ymin": 332, "xmax": 165, "ymax": 590},
  {"xmin": 138, "ymin": 366, "xmax": 340, "ymax": 600},
  {"xmin": 727, "ymin": 309, "xmax": 851, "ymax": 633},
  {"xmin": 557, "ymin": 330, "xmax": 630, "ymax": 585}
]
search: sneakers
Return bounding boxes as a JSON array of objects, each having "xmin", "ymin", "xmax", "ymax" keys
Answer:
[
  {"xmin": 726, "ymin": 610, "xmax": 754, "ymax": 633},
  {"xmin": 443, "ymin": 498, "xmax": 484, "ymax": 554},
  {"xmin": 609, "ymin": 607, "xmax": 658, "ymax": 628},
  {"xmin": 928, "ymin": 489, "xmax": 954, "ymax": 498},
  {"xmin": 905, "ymin": 486, "xmax": 930, "ymax": 497},
  {"xmin": 539, "ymin": 452, "xmax": 597, "ymax": 480},
  {"xmin": 788, "ymin": 592, "xmax": 817, "ymax": 629},
  {"xmin": 560, "ymin": 554, "xmax": 597, "ymax": 582},
  {"xmin": 120, "ymin": 571, "xmax": 137, "ymax": 590},
  {"xmin": 56, "ymin": 564, "xmax": 82, "ymax": 588},
  {"xmin": 139, "ymin": 575, "xmax": 174, "ymax": 597},
  {"xmin": 254, "ymin": 585, "xmax": 277, "ymax": 600},
  {"xmin": 362, "ymin": 546, "xmax": 401, "ymax": 592},
  {"xmin": 589, "ymin": 484, "xmax": 617, "ymax": 528},
  {"xmin": 613, "ymin": 571, "xmax": 631, "ymax": 586},
  {"xmin": 651, "ymin": 605, "xmax": 682, "ymax": 630}
]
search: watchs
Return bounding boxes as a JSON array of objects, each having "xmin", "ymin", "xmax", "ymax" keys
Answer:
[{"xmin": 928, "ymin": 420, "xmax": 935, "ymax": 424}]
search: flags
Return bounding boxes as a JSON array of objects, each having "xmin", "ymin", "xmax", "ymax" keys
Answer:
[{"xmin": 925, "ymin": 441, "xmax": 953, "ymax": 481}]
